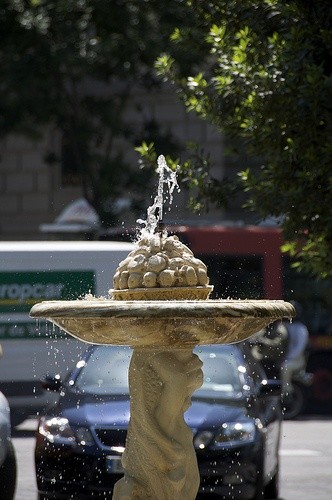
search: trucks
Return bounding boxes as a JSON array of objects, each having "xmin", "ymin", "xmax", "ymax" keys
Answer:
[{"xmin": 1, "ymin": 240, "xmax": 138, "ymax": 428}]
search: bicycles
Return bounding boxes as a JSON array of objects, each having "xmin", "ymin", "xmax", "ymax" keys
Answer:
[{"xmin": 265, "ymin": 348, "xmax": 314, "ymax": 421}]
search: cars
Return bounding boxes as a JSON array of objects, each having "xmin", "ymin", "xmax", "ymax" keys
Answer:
[{"xmin": 34, "ymin": 342, "xmax": 287, "ymax": 500}]
